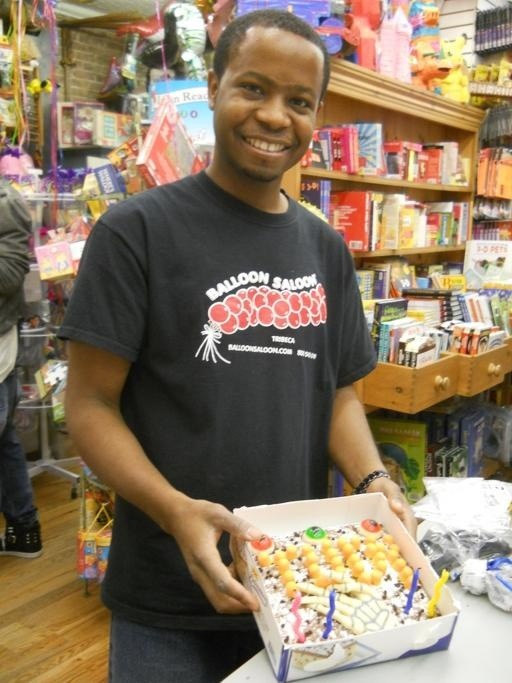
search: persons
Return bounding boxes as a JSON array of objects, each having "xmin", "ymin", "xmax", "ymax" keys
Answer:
[
  {"xmin": 57, "ymin": 8, "xmax": 420, "ymax": 682},
  {"xmin": 1, "ymin": 179, "xmax": 49, "ymax": 557}
]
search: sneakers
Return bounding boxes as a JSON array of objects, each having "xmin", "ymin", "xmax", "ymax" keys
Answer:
[{"xmin": 0, "ymin": 521, "xmax": 44, "ymax": 558}]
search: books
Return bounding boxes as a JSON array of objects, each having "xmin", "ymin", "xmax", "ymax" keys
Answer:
[
  {"xmin": 464, "ymin": 106, "xmax": 511, "ymax": 291},
  {"xmin": 327, "ymin": 257, "xmax": 512, "ymax": 505},
  {"xmin": 294, "ymin": 105, "xmax": 470, "ymax": 252}
]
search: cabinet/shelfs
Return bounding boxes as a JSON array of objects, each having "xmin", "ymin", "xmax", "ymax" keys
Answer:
[{"xmin": 204, "ymin": 46, "xmax": 511, "ymax": 477}]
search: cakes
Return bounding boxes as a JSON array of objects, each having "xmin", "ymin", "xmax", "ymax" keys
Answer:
[{"xmin": 242, "ymin": 517, "xmax": 452, "ymax": 644}]
